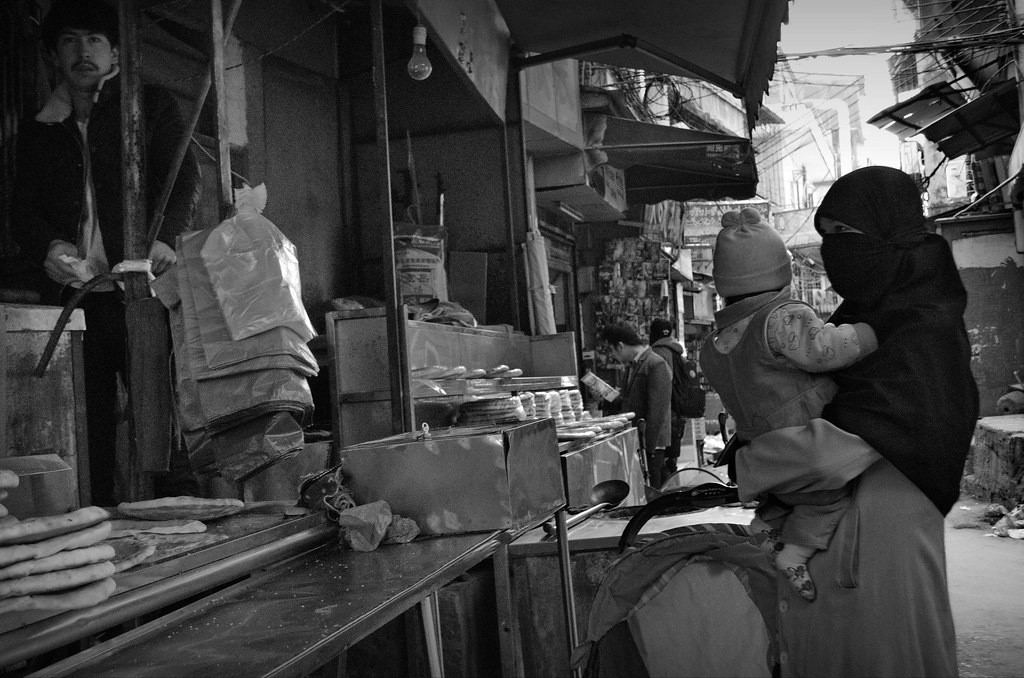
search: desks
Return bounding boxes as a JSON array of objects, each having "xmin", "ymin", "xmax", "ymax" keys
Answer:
[{"xmin": 0, "ymin": 502, "xmax": 581, "ymax": 678}]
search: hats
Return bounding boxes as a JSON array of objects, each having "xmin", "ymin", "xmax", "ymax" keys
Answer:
[
  {"xmin": 650, "ymin": 318, "xmax": 671, "ymax": 338},
  {"xmin": 43, "ymin": 0, "xmax": 120, "ymax": 47},
  {"xmin": 713, "ymin": 207, "xmax": 793, "ymax": 297}
]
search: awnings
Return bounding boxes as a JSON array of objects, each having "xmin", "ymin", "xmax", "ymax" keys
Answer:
[
  {"xmin": 583, "ymin": 111, "xmax": 759, "ymax": 206},
  {"xmin": 870, "ymin": 76, "xmax": 1024, "ymax": 161},
  {"xmin": 495, "ymin": 0, "xmax": 791, "ymax": 140}
]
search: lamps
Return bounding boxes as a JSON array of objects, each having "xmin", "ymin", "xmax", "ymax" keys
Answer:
[{"xmin": 408, "ymin": 0, "xmax": 432, "ymax": 80}]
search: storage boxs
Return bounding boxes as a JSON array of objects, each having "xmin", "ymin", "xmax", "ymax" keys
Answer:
[{"xmin": 339, "ymin": 417, "xmax": 567, "ymax": 537}]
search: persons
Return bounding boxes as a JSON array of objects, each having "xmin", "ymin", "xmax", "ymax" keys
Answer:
[
  {"xmin": 9, "ymin": 0, "xmax": 204, "ymax": 506},
  {"xmin": 699, "ymin": 208, "xmax": 879, "ymax": 602},
  {"xmin": 649, "ymin": 319, "xmax": 687, "ymax": 474},
  {"xmin": 588, "ymin": 323, "xmax": 674, "ymax": 488},
  {"xmin": 777, "ymin": 165, "xmax": 981, "ymax": 678}
]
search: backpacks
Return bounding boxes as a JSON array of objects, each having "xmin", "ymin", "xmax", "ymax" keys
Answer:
[{"xmin": 670, "ymin": 350, "xmax": 706, "ymax": 418}]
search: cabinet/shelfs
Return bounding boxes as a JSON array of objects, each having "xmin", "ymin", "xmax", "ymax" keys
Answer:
[{"xmin": 508, "ymin": 466, "xmax": 757, "ymax": 678}]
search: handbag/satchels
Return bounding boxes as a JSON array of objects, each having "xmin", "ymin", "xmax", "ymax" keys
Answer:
[{"xmin": 154, "ymin": 214, "xmax": 319, "ymax": 483}]
